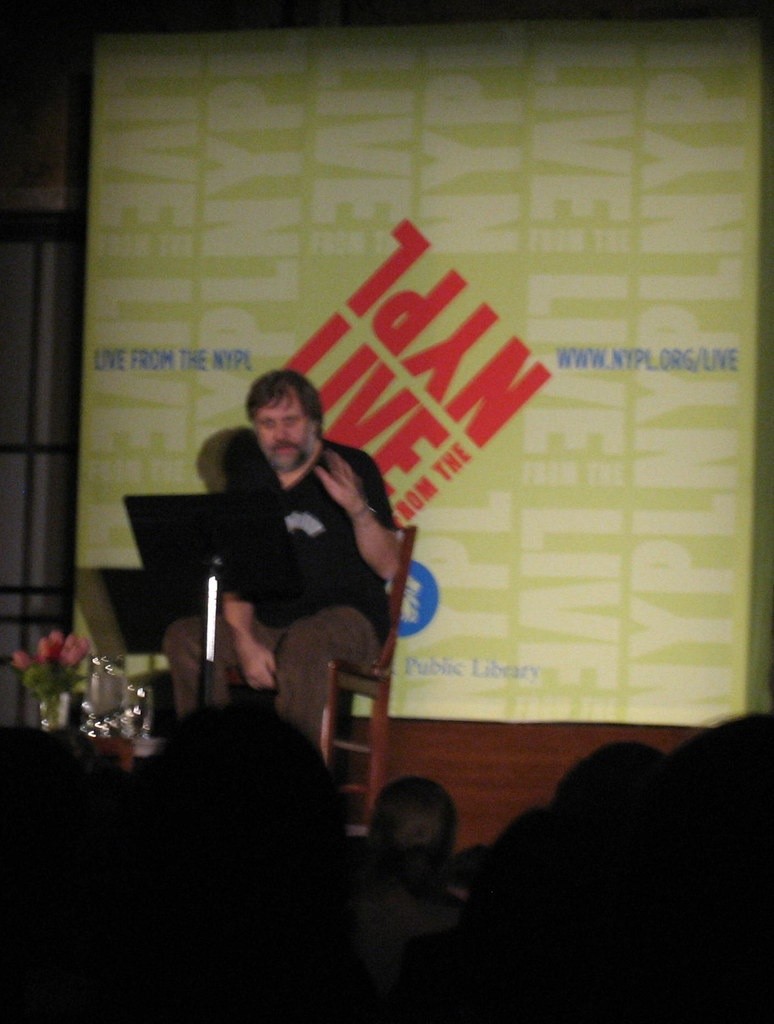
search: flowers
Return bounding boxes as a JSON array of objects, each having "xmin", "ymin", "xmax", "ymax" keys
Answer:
[{"xmin": 11, "ymin": 630, "xmax": 92, "ymax": 729}]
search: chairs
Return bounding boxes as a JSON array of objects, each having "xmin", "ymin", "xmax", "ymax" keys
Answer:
[{"xmin": 217, "ymin": 523, "xmax": 416, "ymax": 826}]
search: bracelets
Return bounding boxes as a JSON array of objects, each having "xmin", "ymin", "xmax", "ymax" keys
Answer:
[{"xmin": 347, "ymin": 506, "xmax": 377, "ymax": 517}]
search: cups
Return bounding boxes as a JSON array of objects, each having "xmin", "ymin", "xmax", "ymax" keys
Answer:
[
  {"xmin": 117, "ymin": 677, "xmax": 153, "ymax": 740},
  {"xmin": 79, "ymin": 654, "xmax": 120, "ymax": 737}
]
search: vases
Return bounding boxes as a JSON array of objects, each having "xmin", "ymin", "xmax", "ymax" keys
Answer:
[
  {"xmin": 98, "ymin": 737, "xmax": 134, "ymax": 772},
  {"xmin": 133, "ymin": 738, "xmax": 161, "ymax": 756},
  {"xmin": 38, "ymin": 693, "xmax": 68, "ymax": 735}
]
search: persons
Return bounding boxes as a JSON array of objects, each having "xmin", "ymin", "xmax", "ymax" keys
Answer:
[
  {"xmin": 163, "ymin": 371, "xmax": 400, "ymax": 744},
  {"xmin": 0, "ymin": 702, "xmax": 774, "ymax": 1024}
]
